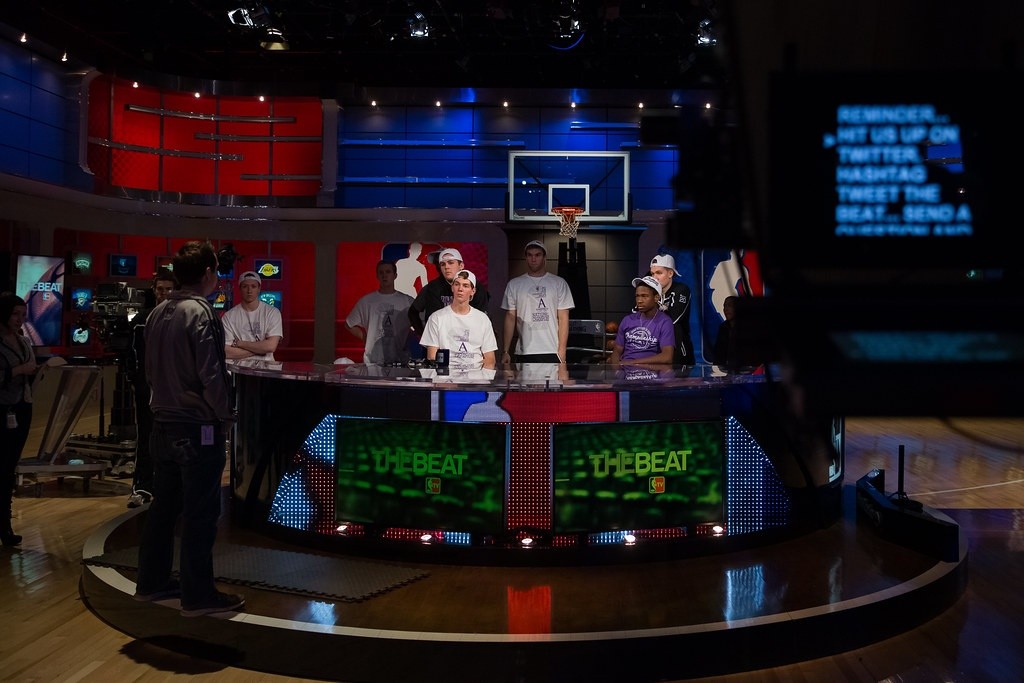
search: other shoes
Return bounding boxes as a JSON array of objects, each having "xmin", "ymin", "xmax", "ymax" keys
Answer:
[
  {"xmin": 182, "ymin": 591, "xmax": 246, "ymax": 617},
  {"xmin": 132, "ymin": 582, "xmax": 185, "ymax": 601},
  {"xmin": 3, "ymin": 535, "xmax": 22, "ymax": 547}
]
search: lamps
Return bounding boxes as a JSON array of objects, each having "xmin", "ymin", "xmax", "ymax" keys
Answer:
[
  {"xmin": 391, "ymin": 0, "xmax": 435, "ymax": 48},
  {"xmin": 679, "ymin": 7, "xmax": 719, "ymax": 50},
  {"xmin": 540, "ymin": 0, "xmax": 590, "ymax": 53},
  {"xmin": 224, "ymin": 1, "xmax": 292, "ymax": 58}
]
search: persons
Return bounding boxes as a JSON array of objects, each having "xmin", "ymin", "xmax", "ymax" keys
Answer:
[
  {"xmin": 344, "ymin": 260, "xmax": 425, "ymax": 363},
  {"xmin": 611, "ymin": 276, "xmax": 675, "ymax": 364},
  {"xmin": 504, "ymin": 362, "xmax": 569, "ymax": 381},
  {"xmin": 0, "ymin": 294, "xmax": 38, "ymax": 544},
  {"xmin": 650, "ymin": 254, "xmax": 695, "ymax": 365},
  {"xmin": 420, "ymin": 270, "xmax": 498, "ymax": 362},
  {"xmin": 611, "ymin": 364, "xmax": 674, "ymax": 384},
  {"xmin": 133, "ymin": 240, "xmax": 247, "ymax": 617},
  {"xmin": 501, "ymin": 240, "xmax": 575, "ymax": 363},
  {"xmin": 407, "ymin": 248, "xmax": 491, "ymax": 335},
  {"xmin": 221, "ymin": 271, "xmax": 283, "ymax": 366},
  {"xmin": 127, "ymin": 268, "xmax": 177, "ymax": 508},
  {"xmin": 712, "ymin": 296, "xmax": 740, "ymax": 368}
]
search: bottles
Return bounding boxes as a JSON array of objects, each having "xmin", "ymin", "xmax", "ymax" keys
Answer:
[
  {"xmin": 392, "ymin": 362, "xmax": 403, "ymax": 381},
  {"xmin": 436, "ymin": 353, "xmax": 444, "ymax": 374},
  {"xmin": 405, "ymin": 356, "xmax": 426, "ymax": 381}
]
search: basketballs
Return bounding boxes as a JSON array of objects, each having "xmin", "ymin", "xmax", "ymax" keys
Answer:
[
  {"xmin": 607, "ymin": 340, "xmax": 615, "ymax": 351},
  {"xmin": 605, "ymin": 321, "xmax": 618, "ymax": 333}
]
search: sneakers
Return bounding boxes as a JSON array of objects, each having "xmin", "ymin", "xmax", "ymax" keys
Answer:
[{"xmin": 126, "ymin": 494, "xmax": 144, "ymax": 507}]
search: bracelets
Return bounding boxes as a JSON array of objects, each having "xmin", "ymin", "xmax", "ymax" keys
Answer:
[
  {"xmin": 503, "ymin": 351, "xmax": 509, "ymax": 353},
  {"xmin": 233, "ymin": 339, "xmax": 238, "ymax": 346}
]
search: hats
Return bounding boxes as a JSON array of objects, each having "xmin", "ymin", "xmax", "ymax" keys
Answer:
[
  {"xmin": 238, "ymin": 270, "xmax": 262, "ymax": 286},
  {"xmin": 632, "ymin": 275, "xmax": 663, "ymax": 296},
  {"xmin": 439, "ymin": 248, "xmax": 463, "ymax": 264},
  {"xmin": 525, "ymin": 240, "xmax": 547, "ymax": 257},
  {"xmin": 451, "ymin": 269, "xmax": 477, "ymax": 288},
  {"xmin": 650, "ymin": 254, "xmax": 682, "ymax": 277}
]
